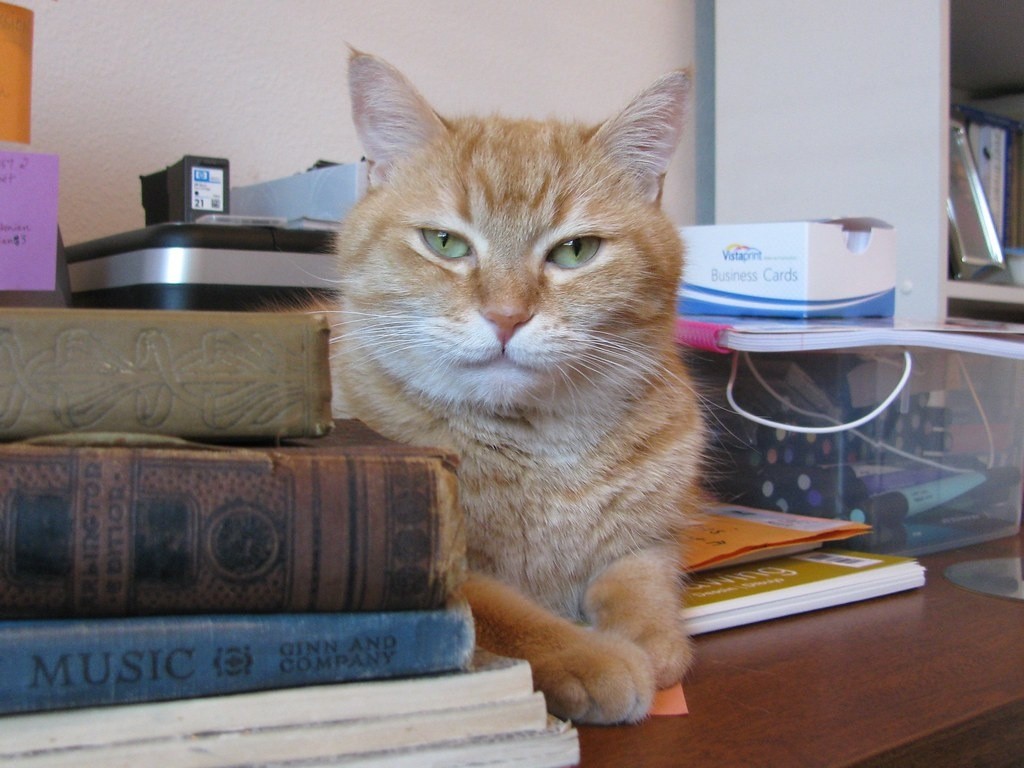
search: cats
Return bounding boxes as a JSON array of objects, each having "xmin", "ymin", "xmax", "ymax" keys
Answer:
[{"xmin": 251, "ymin": 43, "xmax": 769, "ymax": 725}]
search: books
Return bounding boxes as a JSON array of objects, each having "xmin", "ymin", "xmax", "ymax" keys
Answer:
[
  {"xmin": 674, "ymin": 314, "xmax": 1024, "ymax": 635},
  {"xmin": 0, "ymin": 308, "xmax": 583, "ymax": 768}
]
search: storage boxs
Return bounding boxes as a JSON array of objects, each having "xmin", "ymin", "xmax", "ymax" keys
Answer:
[
  {"xmin": 678, "ymin": 345, "xmax": 1024, "ymax": 560},
  {"xmin": 674, "ymin": 215, "xmax": 897, "ymax": 320}
]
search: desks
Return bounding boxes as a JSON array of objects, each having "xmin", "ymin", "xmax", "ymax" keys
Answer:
[{"xmin": 580, "ymin": 518, "xmax": 1024, "ymax": 768}]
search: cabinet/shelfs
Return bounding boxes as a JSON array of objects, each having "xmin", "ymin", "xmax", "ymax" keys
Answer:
[{"xmin": 712, "ymin": 0, "xmax": 1024, "ymax": 324}]
